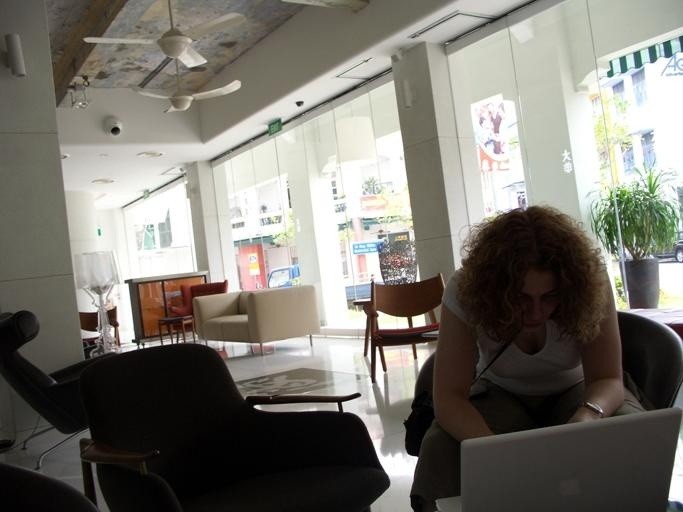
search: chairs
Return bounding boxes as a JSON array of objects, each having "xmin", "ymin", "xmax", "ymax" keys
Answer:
[
  {"xmin": 66, "ymin": 339, "xmax": 392, "ymax": 510},
  {"xmin": 74, "ymin": 305, "xmax": 125, "ymax": 349},
  {"xmin": 363, "ymin": 272, "xmax": 446, "ymax": 381},
  {"xmin": 407, "ymin": 308, "xmax": 683, "ymax": 511},
  {"xmin": 169, "ymin": 283, "xmax": 198, "ymax": 343},
  {"xmin": 1, "ymin": 304, "xmax": 126, "ymax": 470},
  {"xmin": 187, "ymin": 279, "xmax": 230, "ymax": 299}
]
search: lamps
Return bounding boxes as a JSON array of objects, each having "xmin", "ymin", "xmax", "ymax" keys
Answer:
[{"xmin": 71, "ymin": 248, "xmax": 133, "ymax": 361}]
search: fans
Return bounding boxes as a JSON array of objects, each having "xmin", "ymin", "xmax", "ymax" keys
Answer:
[
  {"xmin": 127, "ymin": 60, "xmax": 246, "ymax": 115},
  {"xmin": 81, "ymin": 0, "xmax": 246, "ymax": 73}
]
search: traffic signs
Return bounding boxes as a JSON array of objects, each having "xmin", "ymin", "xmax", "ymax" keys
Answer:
[{"xmin": 353, "ymin": 240, "xmax": 385, "ymax": 255}]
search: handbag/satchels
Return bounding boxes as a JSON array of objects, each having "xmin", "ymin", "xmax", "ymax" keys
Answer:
[{"xmin": 403, "ymin": 350, "xmax": 435, "ymax": 456}]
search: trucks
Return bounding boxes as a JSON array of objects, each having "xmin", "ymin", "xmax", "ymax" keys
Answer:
[{"xmin": 267, "ymin": 265, "xmax": 372, "ymax": 312}]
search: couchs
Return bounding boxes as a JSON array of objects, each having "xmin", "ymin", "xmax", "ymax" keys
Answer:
[{"xmin": 188, "ymin": 283, "xmax": 322, "ymax": 355}]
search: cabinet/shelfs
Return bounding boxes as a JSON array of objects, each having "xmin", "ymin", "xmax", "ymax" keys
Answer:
[{"xmin": 124, "ymin": 271, "xmax": 213, "ymax": 349}]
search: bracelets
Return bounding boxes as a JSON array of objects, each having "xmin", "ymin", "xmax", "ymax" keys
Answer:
[{"xmin": 576, "ymin": 399, "xmax": 605, "ymax": 419}]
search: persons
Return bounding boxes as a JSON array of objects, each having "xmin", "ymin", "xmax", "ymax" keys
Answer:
[
  {"xmin": 472, "ymin": 99, "xmax": 513, "ymax": 160},
  {"xmin": 407, "ymin": 201, "xmax": 649, "ymax": 511}
]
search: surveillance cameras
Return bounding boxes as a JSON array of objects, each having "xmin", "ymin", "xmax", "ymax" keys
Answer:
[
  {"xmin": 295, "ymin": 101, "xmax": 304, "ymax": 107},
  {"xmin": 106, "ymin": 117, "xmax": 122, "ymax": 136}
]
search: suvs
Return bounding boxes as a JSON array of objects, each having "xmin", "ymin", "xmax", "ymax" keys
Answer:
[{"xmin": 644, "ymin": 225, "xmax": 683, "ymax": 265}]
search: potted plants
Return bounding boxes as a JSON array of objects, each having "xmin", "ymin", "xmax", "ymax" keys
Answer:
[{"xmin": 583, "ymin": 156, "xmax": 683, "ymax": 309}]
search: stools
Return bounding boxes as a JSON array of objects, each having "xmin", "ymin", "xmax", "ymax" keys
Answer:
[
  {"xmin": 0, "ymin": 458, "xmax": 98, "ymax": 511},
  {"xmin": 158, "ymin": 316, "xmax": 196, "ymax": 345}
]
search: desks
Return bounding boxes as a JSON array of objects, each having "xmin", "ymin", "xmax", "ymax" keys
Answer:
[
  {"xmin": 421, "ymin": 329, "xmax": 441, "ymax": 338},
  {"xmin": 350, "ymin": 295, "xmax": 440, "ymax": 373}
]
